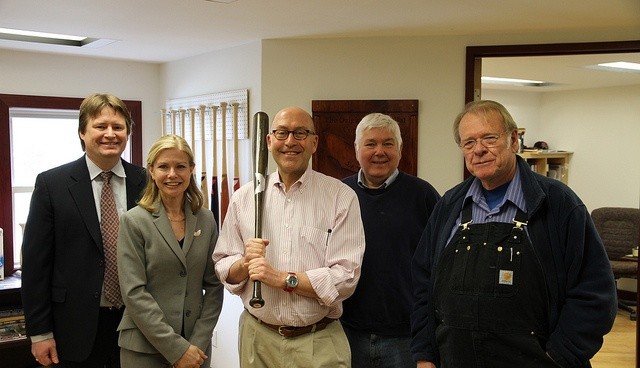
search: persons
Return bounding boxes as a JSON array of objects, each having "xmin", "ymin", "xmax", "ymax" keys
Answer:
[
  {"xmin": 212, "ymin": 106, "xmax": 367, "ymax": 368},
  {"xmin": 407, "ymin": 99, "xmax": 618, "ymax": 367},
  {"xmin": 21, "ymin": 94, "xmax": 153, "ymax": 368},
  {"xmin": 116, "ymin": 134, "xmax": 224, "ymax": 368},
  {"xmin": 333, "ymin": 112, "xmax": 445, "ymax": 368}
]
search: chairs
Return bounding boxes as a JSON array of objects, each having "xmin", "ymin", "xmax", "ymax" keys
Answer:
[{"xmin": 590, "ymin": 206, "xmax": 640, "ymax": 321}]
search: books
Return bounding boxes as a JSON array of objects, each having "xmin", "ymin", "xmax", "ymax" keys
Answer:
[
  {"xmin": 0, "ymin": 308, "xmax": 27, "ymax": 341},
  {"xmin": 530, "ymin": 164, "xmax": 538, "ymax": 173},
  {"xmin": 548, "ymin": 163, "xmax": 562, "ymax": 183}
]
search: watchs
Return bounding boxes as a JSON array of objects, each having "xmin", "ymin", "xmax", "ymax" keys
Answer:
[{"xmin": 283, "ymin": 272, "xmax": 299, "ymax": 292}]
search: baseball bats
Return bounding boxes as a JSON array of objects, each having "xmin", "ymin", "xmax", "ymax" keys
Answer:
[
  {"xmin": 218, "ymin": 102, "xmax": 230, "ymax": 231},
  {"xmin": 209, "ymin": 105, "xmax": 219, "ymax": 235},
  {"xmin": 159, "ymin": 108, "xmax": 167, "ymax": 138},
  {"xmin": 198, "ymin": 104, "xmax": 209, "ymax": 212},
  {"xmin": 170, "ymin": 109, "xmax": 176, "ymax": 135},
  {"xmin": 179, "ymin": 108, "xmax": 185, "ymax": 140},
  {"xmin": 249, "ymin": 112, "xmax": 269, "ymax": 309},
  {"xmin": 229, "ymin": 103, "xmax": 239, "ymax": 193},
  {"xmin": 188, "ymin": 108, "xmax": 196, "ymax": 186}
]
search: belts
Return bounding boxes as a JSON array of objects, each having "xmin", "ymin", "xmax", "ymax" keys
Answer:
[
  {"xmin": 101, "ymin": 305, "xmax": 125, "ymax": 311},
  {"xmin": 243, "ymin": 306, "xmax": 337, "ymax": 339}
]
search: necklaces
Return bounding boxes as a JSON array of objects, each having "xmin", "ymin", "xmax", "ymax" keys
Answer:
[{"xmin": 166, "ymin": 217, "xmax": 185, "ymax": 222}]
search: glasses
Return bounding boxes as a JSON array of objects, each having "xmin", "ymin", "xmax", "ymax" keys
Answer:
[
  {"xmin": 462, "ymin": 132, "xmax": 511, "ymax": 154},
  {"xmin": 270, "ymin": 129, "xmax": 317, "ymax": 140}
]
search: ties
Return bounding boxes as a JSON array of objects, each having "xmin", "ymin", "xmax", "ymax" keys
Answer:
[{"xmin": 99, "ymin": 171, "xmax": 125, "ymax": 308}]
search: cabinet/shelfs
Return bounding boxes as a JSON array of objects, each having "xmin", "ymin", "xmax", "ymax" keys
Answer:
[{"xmin": 517, "ymin": 148, "xmax": 574, "ymax": 184}]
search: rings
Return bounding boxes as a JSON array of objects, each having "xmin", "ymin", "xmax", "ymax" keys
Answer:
[{"xmin": 36, "ymin": 358, "xmax": 39, "ymax": 361}]
